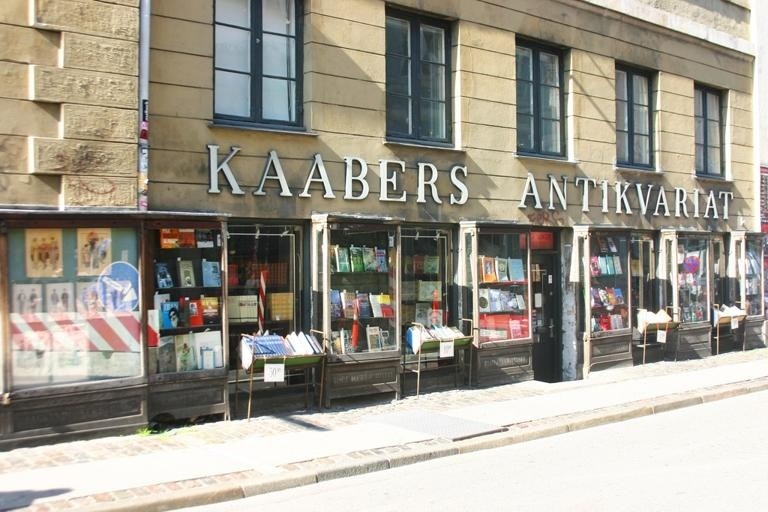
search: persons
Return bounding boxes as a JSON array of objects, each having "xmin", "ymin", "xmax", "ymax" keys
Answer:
[
  {"xmin": 80, "ymin": 232, "xmax": 107, "ymax": 269},
  {"xmin": 16, "ymin": 289, "xmax": 39, "ymax": 312},
  {"xmin": 30, "ymin": 236, "xmax": 59, "ymax": 273},
  {"xmin": 77, "ymin": 284, "xmax": 100, "ymax": 311},
  {"xmin": 50, "ymin": 287, "xmax": 70, "ymax": 312}
]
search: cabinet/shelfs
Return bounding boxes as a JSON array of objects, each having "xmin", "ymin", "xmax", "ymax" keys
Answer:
[
  {"xmin": 328, "ymin": 223, "xmax": 398, "ymax": 363},
  {"xmin": 229, "ymin": 255, "xmax": 297, "ymax": 374},
  {"xmin": 479, "ymin": 228, "xmax": 532, "ymax": 349},
  {"xmin": 591, "ymin": 231, "xmax": 632, "ymax": 338},
  {"xmin": 145, "ymin": 220, "xmax": 228, "ymax": 384}
]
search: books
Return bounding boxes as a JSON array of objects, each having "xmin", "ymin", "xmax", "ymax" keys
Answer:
[
  {"xmin": 230, "ymin": 263, "xmax": 322, "ymax": 372},
  {"xmin": 154, "ymin": 227, "xmax": 223, "ymax": 373},
  {"xmin": 590, "ymin": 236, "xmax": 624, "ymax": 332},
  {"xmin": 478, "ymin": 255, "xmax": 529, "ymax": 344},
  {"xmin": 329, "ymin": 244, "xmax": 393, "ymax": 354},
  {"xmin": 401, "ymin": 254, "xmax": 464, "ymax": 354}
]
